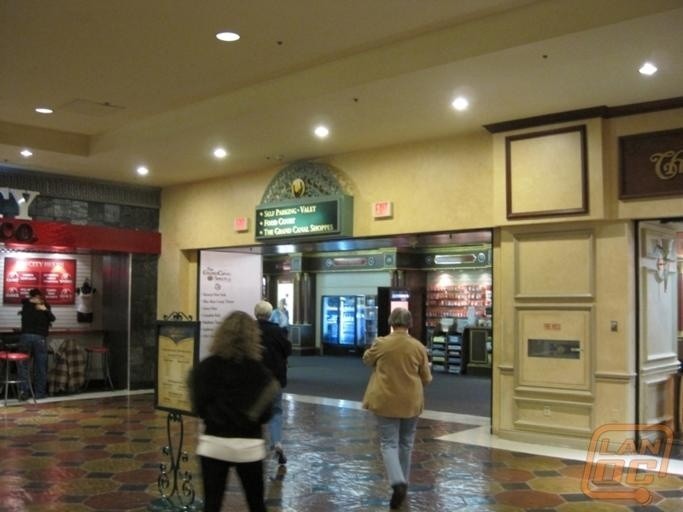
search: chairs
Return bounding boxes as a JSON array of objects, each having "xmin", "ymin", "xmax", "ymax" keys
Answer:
[{"xmin": 0, "ymin": 341, "xmax": 37, "ymax": 407}]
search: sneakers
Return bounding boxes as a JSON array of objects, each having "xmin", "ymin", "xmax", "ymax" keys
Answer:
[
  {"xmin": 390, "ymin": 484, "xmax": 407, "ymax": 509},
  {"xmin": 275, "ymin": 447, "xmax": 287, "ymax": 464}
]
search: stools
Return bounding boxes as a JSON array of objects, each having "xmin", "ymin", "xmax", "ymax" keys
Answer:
[{"xmin": 84, "ymin": 346, "xmax": 113, "ymax": 391}]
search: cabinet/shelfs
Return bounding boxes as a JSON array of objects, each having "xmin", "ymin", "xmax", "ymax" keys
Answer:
[{"xmin": 430, "ymin": 323, "xmax": 468, "ymax": 375}]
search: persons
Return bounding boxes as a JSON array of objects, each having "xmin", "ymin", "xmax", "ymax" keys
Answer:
[
  {"xmin": 75, "ymin": 280, "xmax": 96, "ymax": 325},
  {"xmin": 186, "ymin": 309, "xmax": 281, "ymax": 512},
  {"xmin": 15, "ymin": 288, "xmax": 56, "ymax": 402},
  {"xmin": 20, "ymin": 293, "xmax": 58, "ymax": 322},
  {"xmin": 269, "ymin": 297, "xmax": 290, "ymax": 341},
  {"xmin": 361, "ymin": 306, "xmax": 432, "ymax": 509},
  {"xmin": 254, "ymin": 300, "xmax": 291, "ymax": 464}
]
